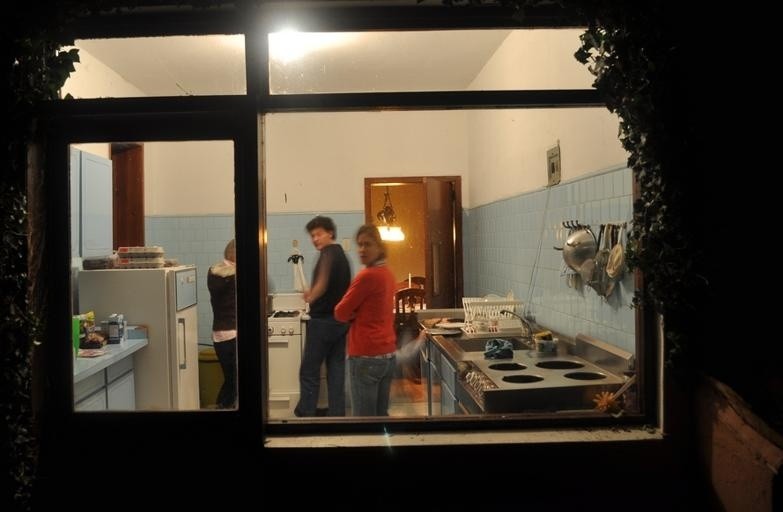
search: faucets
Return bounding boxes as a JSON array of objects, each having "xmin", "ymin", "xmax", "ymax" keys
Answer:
[{"xmin": 500, "ymin": 310, "xmax": 533, "ymax": 340}]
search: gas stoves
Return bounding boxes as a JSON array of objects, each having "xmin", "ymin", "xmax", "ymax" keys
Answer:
[
  {"xmin": 267, "ymin": 309, "xmax": 303, "ymax": 319},
  {"xmin": 469, "ymin": 355, "xmax": 625, "ymax": 392}
]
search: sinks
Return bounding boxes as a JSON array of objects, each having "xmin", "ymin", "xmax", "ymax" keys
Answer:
[{"xmin": 449, "ymin": 335, "xmax": 532, "ymax": 355}]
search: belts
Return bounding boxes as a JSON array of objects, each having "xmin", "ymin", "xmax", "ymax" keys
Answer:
[{"xmin": 356, "ymin": 351, "xmax": 396, "ymax": 361}]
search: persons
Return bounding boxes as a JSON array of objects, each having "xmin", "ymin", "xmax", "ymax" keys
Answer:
[
  {"xmin": 332, "ymin": 224, "xmax": 397, "ymax": 416},
  {"xmin": 286, "ymin": 216, "xmax": 352, "ymax": 419},
  {"xmin": 207, "ymin": 238, "xmax": 237, "ymax": 409}
]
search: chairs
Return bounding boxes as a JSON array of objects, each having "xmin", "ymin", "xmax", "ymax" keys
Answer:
[{"xmin": 392, "ymin": 276, "xmax": 426, "ymax": 380}]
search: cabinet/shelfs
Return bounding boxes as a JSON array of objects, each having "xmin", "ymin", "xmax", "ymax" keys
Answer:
[
  {"xmin": 73, "ymin": 355, "xmax": 136, "ymax": 411},
  {"xmin": 418, "ymin": 327, "xmax": 455, "ymax": 416}
]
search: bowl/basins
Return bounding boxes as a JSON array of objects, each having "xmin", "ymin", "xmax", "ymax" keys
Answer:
[{"xmin": 561, "ymin": 228, "xmax": 597, "ymax": 272}]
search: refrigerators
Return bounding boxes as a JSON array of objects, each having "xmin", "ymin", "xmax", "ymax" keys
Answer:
[{"xmin": 72, "ymin": 263, "xmax": 199, "ymax": 412}]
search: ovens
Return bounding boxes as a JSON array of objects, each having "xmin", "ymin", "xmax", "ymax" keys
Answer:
[{"xmin": 267, "ymin": 335, "xmax": 301, "ymax": 395}]
